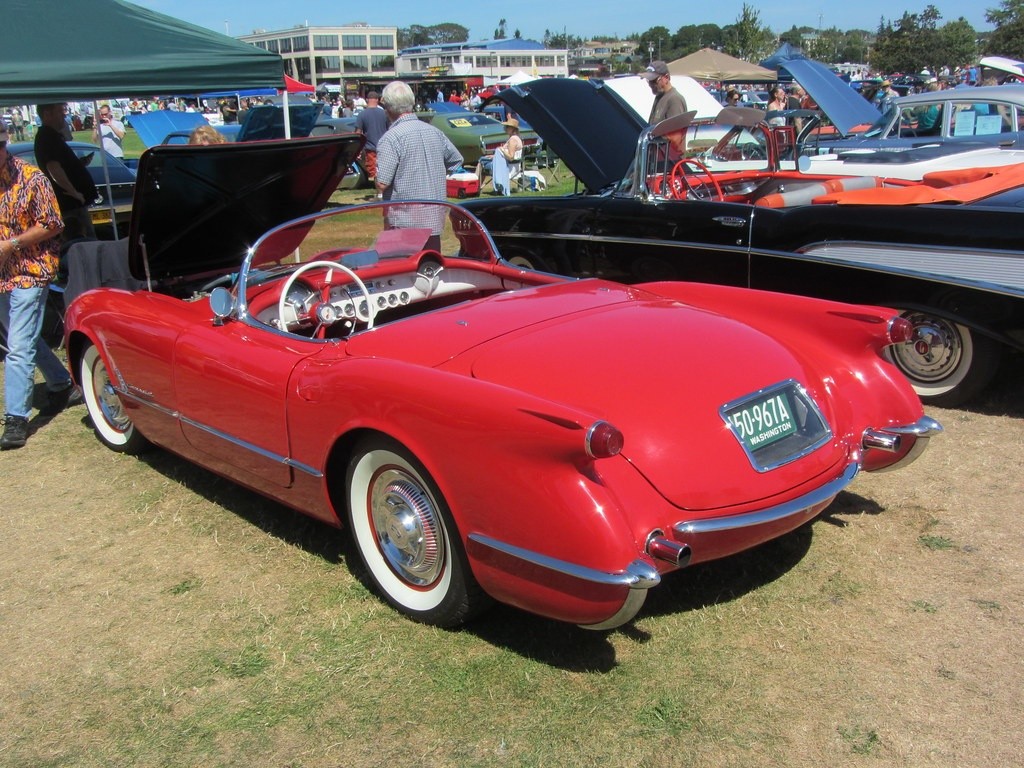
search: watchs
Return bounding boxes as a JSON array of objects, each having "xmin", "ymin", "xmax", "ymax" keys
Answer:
[{"xmin": 11, "ymin": 238, "xmax": 23, "ymax": 251}]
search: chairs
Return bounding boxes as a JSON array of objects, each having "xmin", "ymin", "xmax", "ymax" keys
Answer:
[
  {"xmin": 753, "ymin": 176, "xmax": 883, "ymax": 210},
  {"xmin": 476, "ymin": 141, "xmax": 562, "ymax": 193}
]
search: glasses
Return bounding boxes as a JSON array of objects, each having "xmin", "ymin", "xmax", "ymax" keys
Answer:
[
  {"xmin": 101, "ymin": 113, "xmax": 108, "ymax": 116},
  {"xmin": 504, "ymin": 126, "xmax": 511, "ymax": 129},
  {"xmin": 733, "ymin": 99, "xmax": 738, "ymax": 101},
  {"xmin": 646, "ymin": 75, "xmax": 663, "ymax": 85}
]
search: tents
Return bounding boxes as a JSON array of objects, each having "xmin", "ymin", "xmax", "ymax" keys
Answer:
[
  {"xmin": 664, "ymin": 47, "xmax": 777, "ymax": 93},
  {"xmin": 497, "ymin": 72, "xmax": 537, "ymax": 86},
  {"xmin": 0, "ymin": 0, "xmax": 303, "ymax": 264},
  {"xmin": 277, "ymin": 73, "xmax": 317, "ymax": 100},
  {"xmin": 197, "ymin": 88, "xmax": 278, "ymax": 108}
]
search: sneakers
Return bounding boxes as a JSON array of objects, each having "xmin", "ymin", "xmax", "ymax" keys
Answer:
[
  {"xmin": 0, "ymin": 415, "xmax": 28, "ymax": 447},
  {"xmin": 46, "ymin": 379, "xmax": 81, "ymax": 403}
]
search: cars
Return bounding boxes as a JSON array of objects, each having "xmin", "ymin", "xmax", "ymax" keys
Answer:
[
  {"xmin": 122, "ymin": 111, "xmax": 367, "ymax": 191},
  {"xmin": 6, "ymin": 141, "xmax": 137, "ymax": 239},
  {"xmin": 407, "ymin": 43, "xmax": 1024, "ymax": 414},
  {"xmin": 62, "ymin": 131, "xmax": 946, "ymax": 636}
]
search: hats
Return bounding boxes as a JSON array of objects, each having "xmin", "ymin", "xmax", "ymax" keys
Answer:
[
  {"xmin": 637, "ymin": 60, "xmax": 668, "ymax": 81},
  {"xmin": 368, "ymin": 92, "xmax": 379, "ymax": 99},
  {"xmin": 502, "ymin": 118, "xmax": 519, "ymax": 129},
  {"xmin": 0, "ymin": 119, "xmax": 9, "ymax": 142}
]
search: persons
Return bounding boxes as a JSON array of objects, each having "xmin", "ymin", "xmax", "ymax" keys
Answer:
[
  {"xmin": 414, "ymin": 83, "xmax": 520, "ymax": 122},
  {"xmin": 0, "ymin": 120, "xmax": 82, "ymax": 447},
  {"xmin": 701, "ymin": 81, "xmax": 808, "ymax": 133},
  {"xmin": 0, "ymin": 93, "xmax": 273, "ymax": 160},
  {"xmin": 34, "ymin": 102, "xmax": 99, "ymax": 241},
  {"xmin": 640, "ymin": 59, "xmax": 689, "ymax": 162},
  {"xmin": 355, "ymin": 91, "xmax": 391, "ymax": 198},
  {"xmin": 842, "ymin": 65, "xmax": 1013, "ymax": 137},
  {"xmin": 723, "ymin": 90, "xmax": 739, "ymax": 108},
  {"xmin": 474, "ymin": 119, "xmax": 523, "ymax": 183},
  {"xmin": 306, "ymin": 91, "xmax": 384, "ymax": 118},
  {"xmin": 373, "ymin": 81, "xmax": 465, "ymax": 257}
]
